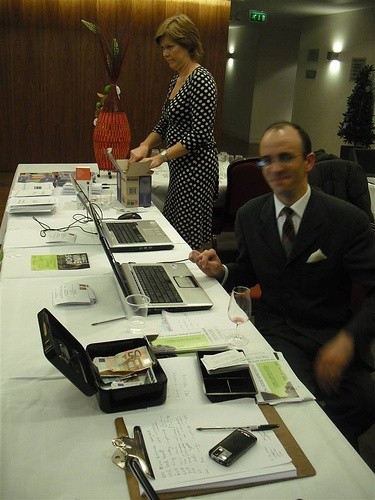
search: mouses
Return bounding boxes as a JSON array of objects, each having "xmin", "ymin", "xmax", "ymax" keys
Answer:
[{"xmin": 117, "ymin": 212, "xmax": 142, "ymax": 219}]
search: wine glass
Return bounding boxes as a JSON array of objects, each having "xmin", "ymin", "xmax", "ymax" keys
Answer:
[{"xmin": 225, "ymin": 286, "xmax": 252, "ymax": 348}]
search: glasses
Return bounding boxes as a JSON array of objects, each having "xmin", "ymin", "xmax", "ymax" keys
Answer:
[{"xmin": 256, "ymin": 153, "xmax": 306, "ymax": 167}]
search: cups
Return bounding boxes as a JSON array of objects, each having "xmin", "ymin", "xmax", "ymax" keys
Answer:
[
  {"xmin": 228, "ymin": 155, "xmax": 243, "ymax": 165},
  {"xmin": 100, "ymin": 190, "xmax": 112, "ymax": 210},
  {"xmin": 151, "ymin": 149, "xmax": 159, "ymax": 156},
  {"xmin": 125, "ymin": 294, "xmax": 151, "ymax": 335}
]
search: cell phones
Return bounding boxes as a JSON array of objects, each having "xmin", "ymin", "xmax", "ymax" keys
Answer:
[{"xmin": 208, "ymin": 428, "xmax": 258, "ymax": 467}]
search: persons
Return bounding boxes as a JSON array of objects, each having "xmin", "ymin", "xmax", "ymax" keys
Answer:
[
  {"xmin": 189, "ymin": 121, "xmax": 374, "ymax": 444},
  {"xmin": 128, "ymin": 13, "xmax": 219, "ymax": 257}
]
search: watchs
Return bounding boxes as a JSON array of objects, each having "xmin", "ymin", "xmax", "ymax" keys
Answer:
[{"xmin": 160, "ymin": 150, "xmax": 169, "ymax": 162}]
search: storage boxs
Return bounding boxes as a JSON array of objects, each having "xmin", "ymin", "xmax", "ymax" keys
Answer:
[
  {"xmin": 105, "ymin": 147, "xmax": 155, "ymax": 208},
  {"xmin": 36, "ymin": 308, "xmax": 168, "ymax": 414}
]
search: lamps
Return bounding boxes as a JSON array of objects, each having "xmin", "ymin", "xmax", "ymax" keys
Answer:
[
  {"xmin": 327, "ymin": 51, "xmax": 343, "ymax": 60},
  {"xmin": 228, "ymin": 53, "xmax": 235, "ymax": 58}
]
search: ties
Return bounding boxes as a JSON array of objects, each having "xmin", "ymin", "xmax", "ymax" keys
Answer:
[{"xmin": 281, "ymin": 207, "xmax": 295, "ymax": 263}]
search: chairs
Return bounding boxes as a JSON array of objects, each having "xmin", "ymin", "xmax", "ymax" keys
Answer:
[
  {"xmin": 212, "ymin": 156, "xmax": 269, "ymax": 253},
  {"xmin": 307, "ymin": 158, "xmax": 375, "ymax": 223}
]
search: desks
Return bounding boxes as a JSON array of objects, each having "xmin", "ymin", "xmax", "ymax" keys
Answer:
[{"xmin": 0, "ymin": 157, "xmax": 375, "ymax": 500}]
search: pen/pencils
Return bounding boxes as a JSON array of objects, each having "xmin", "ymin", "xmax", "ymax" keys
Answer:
[
  {"xmin": 91, "ymin": 316, "xmax": 126, "ymax": 327},
  {"xmin": 196, "ymin": 423, "xmax": 281, "ymax": 432}
]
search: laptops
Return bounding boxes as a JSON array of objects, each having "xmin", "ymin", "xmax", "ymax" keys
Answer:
[
  {"xmin": 74, "ymin": 178, "xmax": 174, "ymax": 254},
  {"xmin": 87, "ymin": 203, "xmax": 213, "ymax": 315}
]
